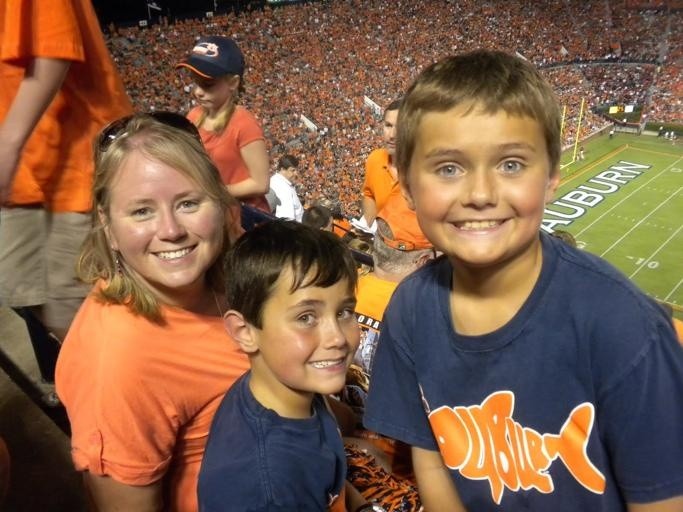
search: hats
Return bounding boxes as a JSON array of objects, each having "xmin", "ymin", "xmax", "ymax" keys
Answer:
[{"xmin": 175, "ymin": 36, "xmax": 244, "ymax": 78}]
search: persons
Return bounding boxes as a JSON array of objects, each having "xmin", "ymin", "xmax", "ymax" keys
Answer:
[
  {"xmin": 197, "ymin": 219, "xmax": 390, "ymax": 511},
  {"xmin": 360, "ymin": 49, "xmax": 683, "ymax": 511},
  {"xmin": 102, "ymin": 0, "xmax": 683, "ymax": 282},
  {"xmin": 54, "ymin": 110, "xmax": 256, "ymax": 512},
  {"xmin": 0, "ymin": 0, "xmax": 137, "ymax": 382}
]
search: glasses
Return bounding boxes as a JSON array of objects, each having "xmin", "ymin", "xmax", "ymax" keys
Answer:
[{"xmin": 99, "ymin": 111, "xmax": 207, "ymax": 153}]
